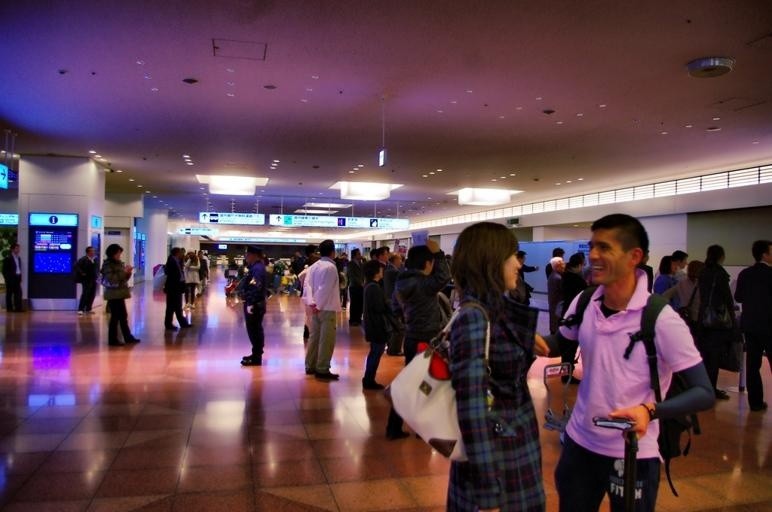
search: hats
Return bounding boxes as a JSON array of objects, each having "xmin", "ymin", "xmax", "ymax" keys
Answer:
[{"xmin": 247, "ymin": 246, "xmax": 262, "ymax": 255}]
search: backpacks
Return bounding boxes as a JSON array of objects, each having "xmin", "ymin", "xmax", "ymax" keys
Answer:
[{"xmin": 559, "ymin": 286, "xmax": 700, "ymax": 496}]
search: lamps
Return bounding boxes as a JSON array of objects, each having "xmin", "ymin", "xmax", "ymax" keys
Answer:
[
  {"xmin": 446, "ymin": 187, "xmax": 525, "ymax": 206},
  {"xmin": 194, "ymin": 174, "xmax": 269, "ymax": 196},
  {"xmin": 686, "ymin": 56, "xmax": 736, "ymax": 78},
  {"xmin": 378, "ymin": 99, "xmax": 387, "ymax": 167},
  {"xmin": 327, "ymin": 181, "xmax": 404, "ymax": 201}
]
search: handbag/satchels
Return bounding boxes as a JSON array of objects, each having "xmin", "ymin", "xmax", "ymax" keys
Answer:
[
  {"xmin": 102, "ymin": 278, "xmax": 119, "ymax": 288},
  {"xmin": 678, "ymin": 306, "xmax": 692, "ymax": 324},
  {"xmin": 702, "ymin": 305, "xmax": 732, "ymax": 329},
  {"xmin": 383, "ymin": 304, "xmax": 494, "ymax": 463}
]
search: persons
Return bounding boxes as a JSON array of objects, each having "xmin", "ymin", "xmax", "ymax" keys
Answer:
[
  {"xmin": 267, "ymin": 251, "xmax": 306, "ymax": 296},
  {"xmin": 644, "ymin": 243, "xmax": 772, "ymax": 412},
  {"xmin": 101, "ymin": 243, "xmax": 141, "ymax": 345},
  {"xmin": 301, "ymin": 239, "xmax": 453, "ymax": 441},
  {"xmin": 236, "ymin": 250, "xmax": 269, "ymax": 366},
  {"xmin": 73, "ymin": 246, "xmax": 98, "ymax": 315},
  {"xmin": 531, "ymin": 213, "xmax": 719, "ymax": 512},
  {"xmin": 437, "ymin": 220, "xmax": 549, "ymax": 510},
  {"xmin": 225, "ymin": 259, "xmax": 248, "ymax": 296},
  {"xmin": 2, "ymin": 243, "xmax": 25, "ymax": 313},
  {"xmin": 512, "ymin": 247, "xmax": 600, "ymax": 385},
  {"xmin": 164, "ymin": 247, "xmax": 211, "ymax": 332}
]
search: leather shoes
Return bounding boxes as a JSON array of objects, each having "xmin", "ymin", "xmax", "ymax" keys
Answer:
[
  {"xmin": 306, "ymin": 370, "xmax": 339, "ymax": 378},
  {"xmin": 241, "ymin": 356, "xmax": 261, "ymax": 365}
]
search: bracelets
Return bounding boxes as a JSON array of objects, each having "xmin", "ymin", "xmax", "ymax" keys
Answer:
[{"xmin": 641, "ymin": 402, "xmax": 658, "ymax": 420}]
